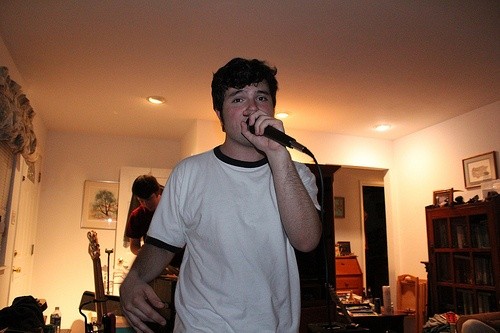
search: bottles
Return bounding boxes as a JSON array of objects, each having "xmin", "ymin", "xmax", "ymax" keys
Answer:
[
  {"xmin": 374, "ymin": 298, "xmax": 381, "ymax": 314},
  {"xmin": 52, "ymin": 307, "xmax": 61, "ymax": 333}
]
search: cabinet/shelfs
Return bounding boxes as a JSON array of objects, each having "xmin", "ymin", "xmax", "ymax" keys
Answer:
[
  {"xmin": 335, "ymin": 255, "xmax": 363, "ymax": 297},
  {"xmin": 425, "ymin": 198, "xmax": 500, "ymax": 317}
]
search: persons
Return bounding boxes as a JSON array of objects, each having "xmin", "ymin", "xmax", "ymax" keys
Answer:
[
  {"xmin": 125, "ymin": 175, "xmax": 162, "ymax": 254},
  {"xmin": 121, "ymin": 58, "xmax": 321, "ymax": 333}
]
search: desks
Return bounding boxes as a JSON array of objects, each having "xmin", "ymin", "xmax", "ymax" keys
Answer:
[{"xmin": 335, "ymin": 297, "xmax": 409, "ymax": 333}]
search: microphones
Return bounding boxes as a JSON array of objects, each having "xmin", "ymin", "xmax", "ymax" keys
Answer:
[{"xmin": 246, "ymin": 117, "xmax": 314, "ymax": 157}]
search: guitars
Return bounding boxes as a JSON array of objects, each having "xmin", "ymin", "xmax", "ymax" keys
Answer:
[{"xmin": 78, "ymin": 230, "xmax": 120, "ymax": 333}]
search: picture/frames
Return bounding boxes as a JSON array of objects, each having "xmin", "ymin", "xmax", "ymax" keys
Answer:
[
  {"xmin": 462, "ymin": 151, "xmax": 497, "ymax": 189},
  {"xmin": 338, "ymin": 242, "xmax": 350, "ymax": 255},
  {"xmin": 432, "ymin": 188, "xmax": 453, "ymax": 206},
  {"xmin": 81, "ymin": 179, "xmax": 119, "ymax": 229},
  {"xmin": 333, "ymin": 196, "xmax": 345, "ymax": 218}
]
